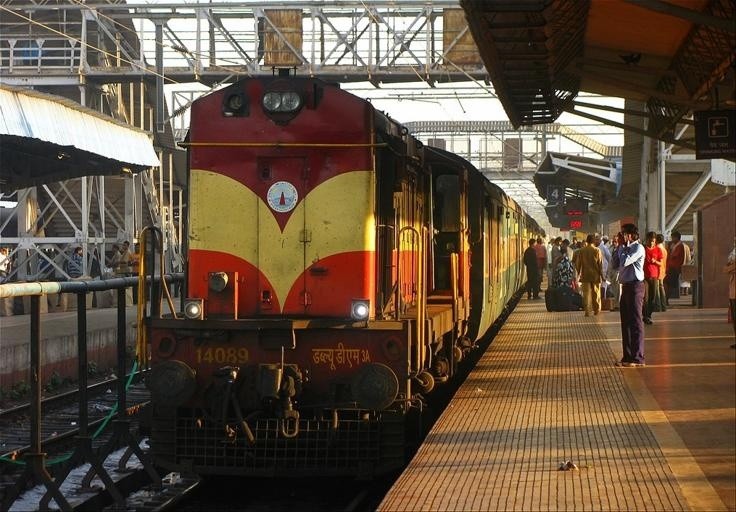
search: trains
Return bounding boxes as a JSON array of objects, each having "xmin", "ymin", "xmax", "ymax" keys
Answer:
[{"xmin": 132, "ymin": 59, "xmax": 547, "ymax": 474}]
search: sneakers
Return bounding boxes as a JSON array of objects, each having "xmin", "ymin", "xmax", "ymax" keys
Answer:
[
  {"xmin": 642, "ymin": 315, "xmax": 654, "ymax": 325},
  {"xmin": 614, "ymin": 357, "xmax": 646, "ymax": 368}
]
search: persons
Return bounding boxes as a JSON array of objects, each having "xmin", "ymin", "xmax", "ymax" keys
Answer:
[
  {"xmin": 106, "ymin": 240, "xmax": 140, "ymax": 308},
  {"xmin": 522, "ymin": 223, "xmax": 691, "ymax": 368},
  {"xmin": 68, "ymin": 247, "xmax": 84, "ymax": 277}
]
